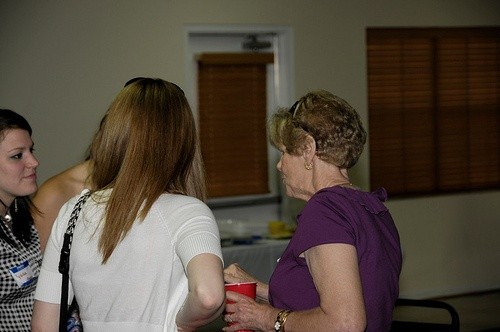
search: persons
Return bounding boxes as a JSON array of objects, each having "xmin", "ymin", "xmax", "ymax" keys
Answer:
[
  {"xmin": 31, "ymin": 155, "xmax": 104, "ymax": 266},
  {"xmin": 0, "ymin": 106, "xmax": 59, "ymax": 332},
  {"xmin": 217, "ymin": 90, "xmax": 404, "ymax": 332},
  {"xmin": 30, "ymin": 77, "xmax": 226, "ymax": 332}
]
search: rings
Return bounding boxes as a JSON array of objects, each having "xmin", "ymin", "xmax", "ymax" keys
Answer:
[{"xmin": 228, "ymin": 314, "xmax": 233, "ymax": 323}]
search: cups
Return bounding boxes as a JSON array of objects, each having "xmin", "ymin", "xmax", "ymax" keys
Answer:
[
  {"xmin": 269, "ymin": 222, "xmax": 286, "ymax": 234},
  {"xmin": 225, "ymin": 282, "xmax": 257, "ymax": 332}
]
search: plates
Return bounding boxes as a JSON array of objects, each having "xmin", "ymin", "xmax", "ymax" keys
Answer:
[{"xmin": 264, "ymin": 233, "xmax": 292, "ymax": 239}]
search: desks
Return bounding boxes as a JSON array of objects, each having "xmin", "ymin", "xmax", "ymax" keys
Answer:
[{"xmin": 220, "ymin": 236, "xmax": 294, "ymax": 285}]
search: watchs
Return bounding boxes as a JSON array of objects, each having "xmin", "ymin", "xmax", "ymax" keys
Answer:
[{"xmin": 274, "ymin": 310, "xmax": 292, "ymax": 332}]
